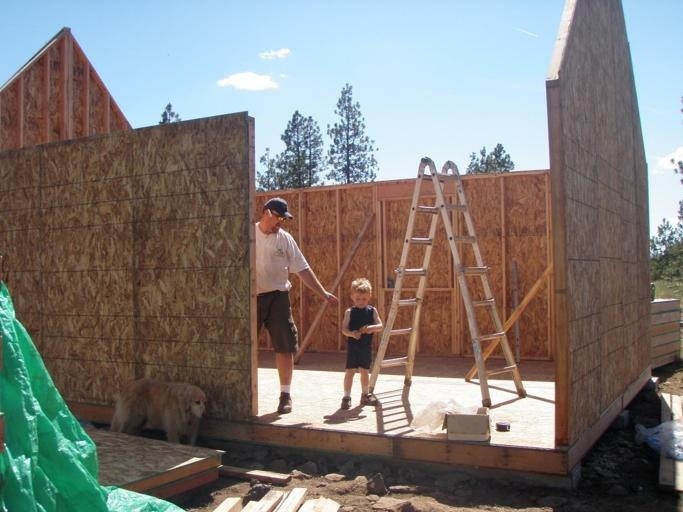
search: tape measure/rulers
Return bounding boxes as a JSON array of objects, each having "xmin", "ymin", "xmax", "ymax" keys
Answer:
[{"xmin": 496, "ymin": 422, "xmax": 511, "ymax": 431}]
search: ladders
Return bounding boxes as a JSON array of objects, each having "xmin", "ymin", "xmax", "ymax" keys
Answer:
[{"xmin": 368, "ymin": 156, "xmax": 527, "ymax": 407}]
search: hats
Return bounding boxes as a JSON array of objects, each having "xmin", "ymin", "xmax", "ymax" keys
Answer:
[{"xmin": 264, "ymin": 198, "xmax": 294, "ymax": 220}]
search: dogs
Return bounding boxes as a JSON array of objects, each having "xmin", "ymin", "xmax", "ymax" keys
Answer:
[{"xmin": 110, "ymin": 379, "xmax": 207, "ymax": 447}]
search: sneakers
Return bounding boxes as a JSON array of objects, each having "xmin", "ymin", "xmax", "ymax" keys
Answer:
[
  {"xmin": 278, "ymin": 392, "xmax": 292, "ymax": 413},
  {"xmin": 341, "ymin": 396, "xmax": 351, "ymax": 409},
  {"xmin": 361, "ymin": 393, "xmax": 378, "ymax": 406}
]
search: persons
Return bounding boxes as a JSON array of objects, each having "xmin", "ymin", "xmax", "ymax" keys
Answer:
[
  {"xmin": 256, "ymin": 196, "xmax": 340, "ymax": 415},
  {"xmin": 339, "ymin": 277, "xmax": 384, "ymax": 410}
]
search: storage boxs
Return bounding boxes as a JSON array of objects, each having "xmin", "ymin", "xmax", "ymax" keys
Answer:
[{"xmin": 442, "ymin": 407, "xmax": 492, "ymax": 442}]
